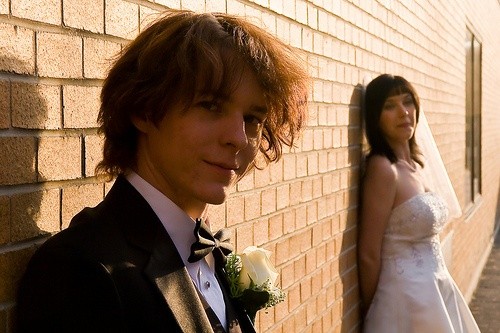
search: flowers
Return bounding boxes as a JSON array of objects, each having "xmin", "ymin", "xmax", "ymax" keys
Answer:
[{"xmin": 224, "ymin": 247, "xmax": 287, "ymax": 327}]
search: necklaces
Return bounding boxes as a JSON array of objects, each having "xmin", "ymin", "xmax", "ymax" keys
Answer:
[{"xmin": 398, "ymin": 159, "xmax": 418, "ymax": 172}]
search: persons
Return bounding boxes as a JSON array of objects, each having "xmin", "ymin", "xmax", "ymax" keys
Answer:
[
  {"xmin": 13, "ymin": 8, "xmax": 310, "ymax": 333},
  {"xmin": 359, "ymin": 72, "xmax": 478, "ymax": 333}
]
